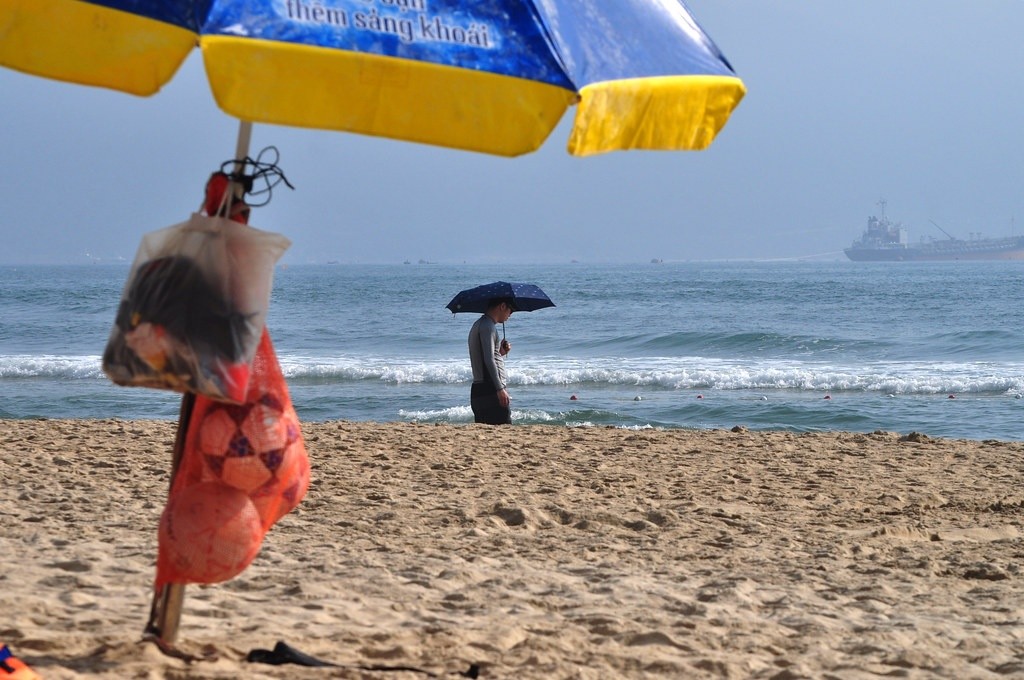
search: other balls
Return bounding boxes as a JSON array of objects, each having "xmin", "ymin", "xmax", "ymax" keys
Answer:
[
  {"xmin": 195, "ymin": 389, "xmax": 312, "ymax": 517},
  {"xmin": 158, "ymin": 481, "xmax": 265, "ymax": 585}
]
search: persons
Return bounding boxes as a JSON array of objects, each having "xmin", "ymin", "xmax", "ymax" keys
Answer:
[{"xmin": 468, "ymin": 297, "xmax": 513, "ymax": 424}]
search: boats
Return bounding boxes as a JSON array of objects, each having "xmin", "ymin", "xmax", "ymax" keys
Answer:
[{"xmin": 844, "ymin": 201, "xmax": 1024, "ymax": 263}]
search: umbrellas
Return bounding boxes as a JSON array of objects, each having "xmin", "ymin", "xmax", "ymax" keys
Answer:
[
  {"xmin": 445, "ymin": 281, "xmax": 557, "ymax": 357},
  {"xmin": 1, "ymin": 0, "xmax": 747, "ymax": 642}
]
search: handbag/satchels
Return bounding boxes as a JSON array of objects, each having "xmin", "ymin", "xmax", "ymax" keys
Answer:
[
  {"xmin": 101, "ymin": 174, "xmax": 291, "ymax": 406},
  {"xmin": 152, "ymin": 171, "xmax": 311, "ymax": 593}
]
